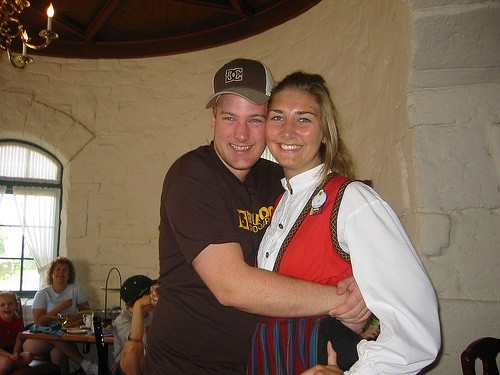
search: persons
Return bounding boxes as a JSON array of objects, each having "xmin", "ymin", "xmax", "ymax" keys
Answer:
[
  {"xmin": 143, "ymin": 58, "xmax": 380, "ymax": 375},
  {"xmin": 150, "ymin": 71, "xmax": 441, "ymax": 375},
  {"xmin": 0, "ymin": 291, "xmax": 33, "ymax": 375},
  {"xmin": 113, "ymin": 276, "xmax": 156, "ymax": 375},
  {"xmin": 23, "ymin": 257, "xmax": 98, "ymax": 375}
]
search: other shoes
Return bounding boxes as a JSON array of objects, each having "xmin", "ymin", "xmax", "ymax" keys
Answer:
[{"xmin": 84, "ymin": 361, "xmax": 98, "ymax": 375}]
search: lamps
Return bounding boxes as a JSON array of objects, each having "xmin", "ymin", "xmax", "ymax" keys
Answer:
[{"xmin": 0, "ymin": 0, "xmax": 60, "ymax": 70}]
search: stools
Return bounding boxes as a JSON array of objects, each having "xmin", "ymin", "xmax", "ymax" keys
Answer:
[{"xmin": 32, "ymin": 353, "xmax": 70, "ymax": 375}]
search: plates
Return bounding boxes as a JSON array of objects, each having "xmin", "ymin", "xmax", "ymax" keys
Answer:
[{"xmin": 67, "ymin": 330, "xmax": 86, "ymax": 333}]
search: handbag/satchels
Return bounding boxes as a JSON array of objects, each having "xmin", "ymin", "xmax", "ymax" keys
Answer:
[{"xmin": 30, "ymin": 320, "xmax": 64, "ymax": 334}]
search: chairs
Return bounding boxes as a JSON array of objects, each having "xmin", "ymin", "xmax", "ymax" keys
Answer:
[{"xmin": 461, "ymin": 337, "xmax": 500, "ymax": 375}]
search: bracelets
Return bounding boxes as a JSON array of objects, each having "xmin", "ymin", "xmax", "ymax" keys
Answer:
[{"xmin": 127, "ymin": 335, "xmax": 142, "ymax": 341}]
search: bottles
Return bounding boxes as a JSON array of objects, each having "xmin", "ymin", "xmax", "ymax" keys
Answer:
[{"xmin": 101, "ymin": 319, "xmax": 112, "ymax": 330}]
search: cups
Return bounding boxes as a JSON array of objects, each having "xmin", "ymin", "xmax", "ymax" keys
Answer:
[{"xmin": 83, "ymin": 314, "xmax": 92, "ymax": 329}]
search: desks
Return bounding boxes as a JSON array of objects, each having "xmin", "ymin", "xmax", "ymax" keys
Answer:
[{"xmin": 17, "ymin": 329, "xmax": 114, "ymax": 375}]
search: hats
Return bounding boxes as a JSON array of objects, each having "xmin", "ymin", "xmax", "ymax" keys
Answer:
[
  {"xmin": 205, "ymin": 58, "xmax": 274, "ymax": 109},
  {"xmin": 120, "ymin": 275, "xmax": 157, "ymax": 305}
]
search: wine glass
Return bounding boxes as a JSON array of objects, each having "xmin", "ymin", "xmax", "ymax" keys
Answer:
[{"xmin": 57, "ymin": 313, "xmax": 68, "ymax": 326}]
